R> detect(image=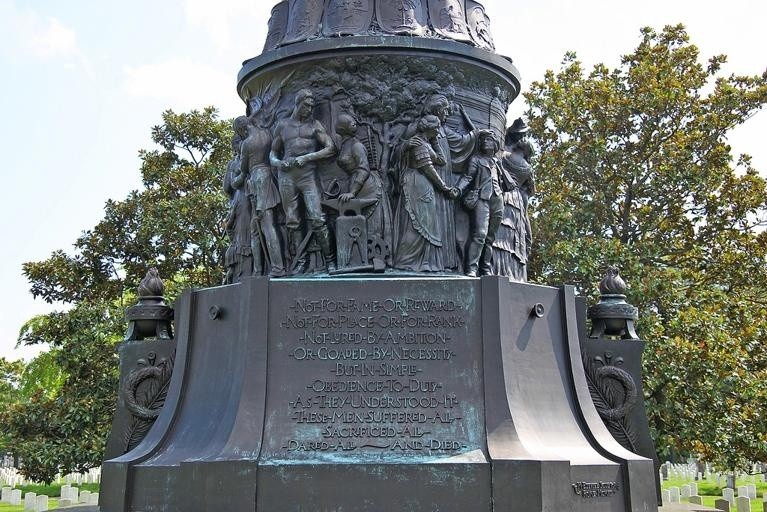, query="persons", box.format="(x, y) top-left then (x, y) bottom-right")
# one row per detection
(221, 89), (536, 283)
